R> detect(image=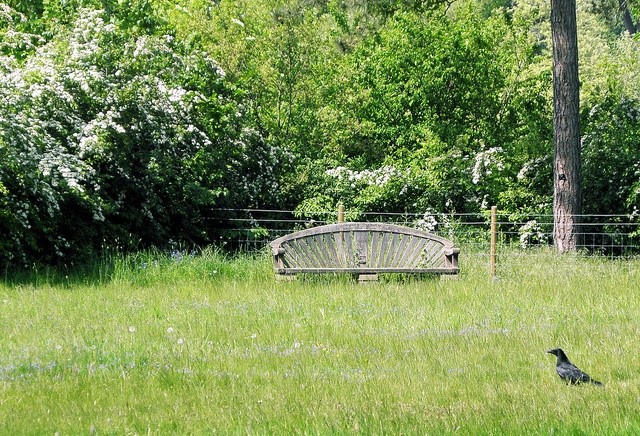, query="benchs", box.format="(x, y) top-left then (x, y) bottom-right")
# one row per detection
(269, 222), (461, 287)
